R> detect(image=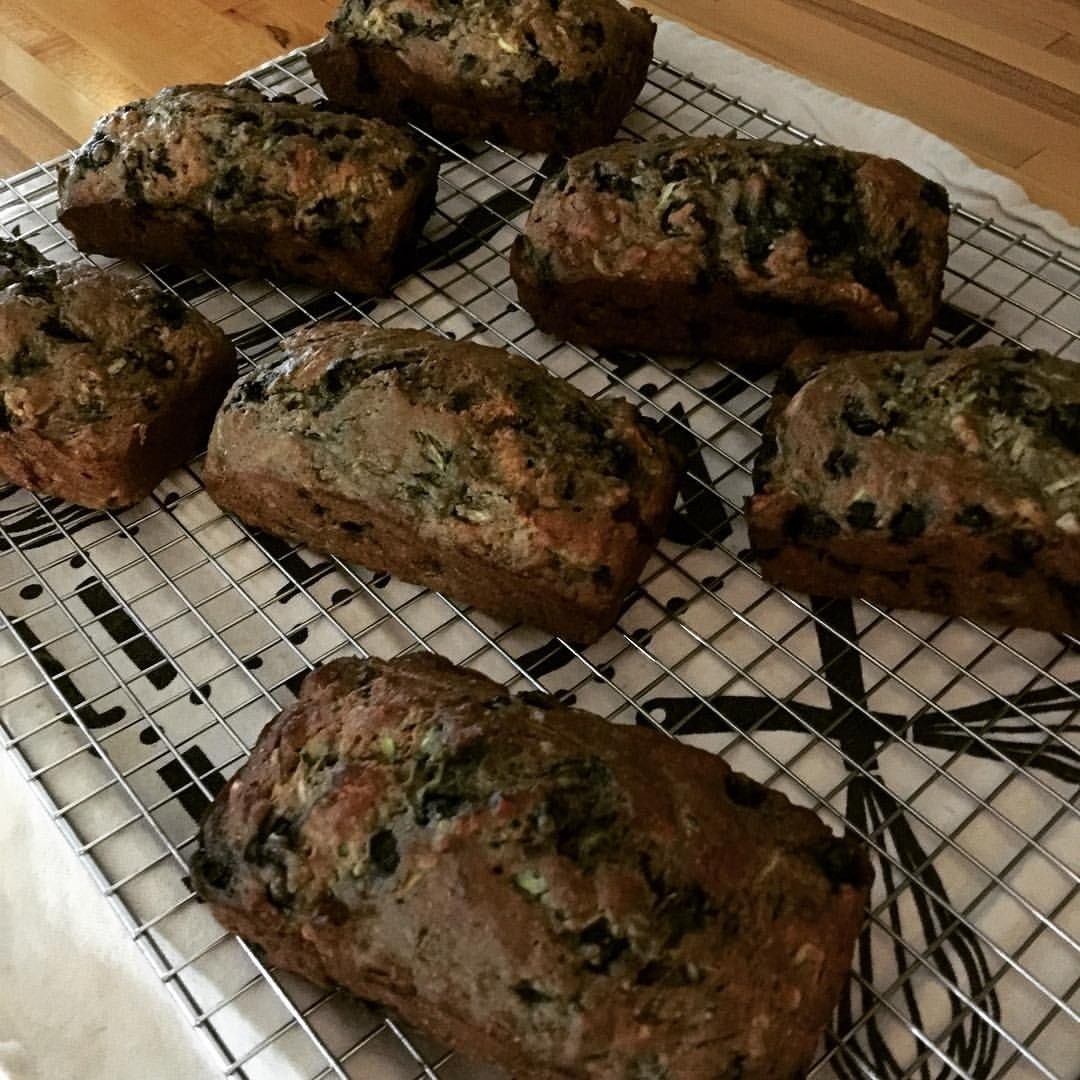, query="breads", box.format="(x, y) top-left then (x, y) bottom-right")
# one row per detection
(0, 0), (1080, 1080)
